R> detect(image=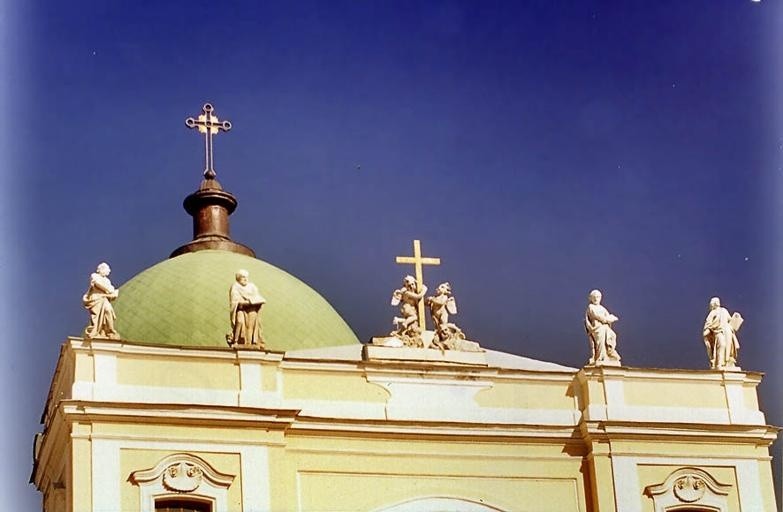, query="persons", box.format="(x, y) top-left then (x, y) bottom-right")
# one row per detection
(80, 257), (121, 341)
(225, 262), (265, 344)
(388, 271), (430, 336)
(700, 295), (745, 371)
(423, 277), (459, 329)
(398, 320), (424, 349)
(429, 323), (481, 352)
(581, 287), (624, 367)
